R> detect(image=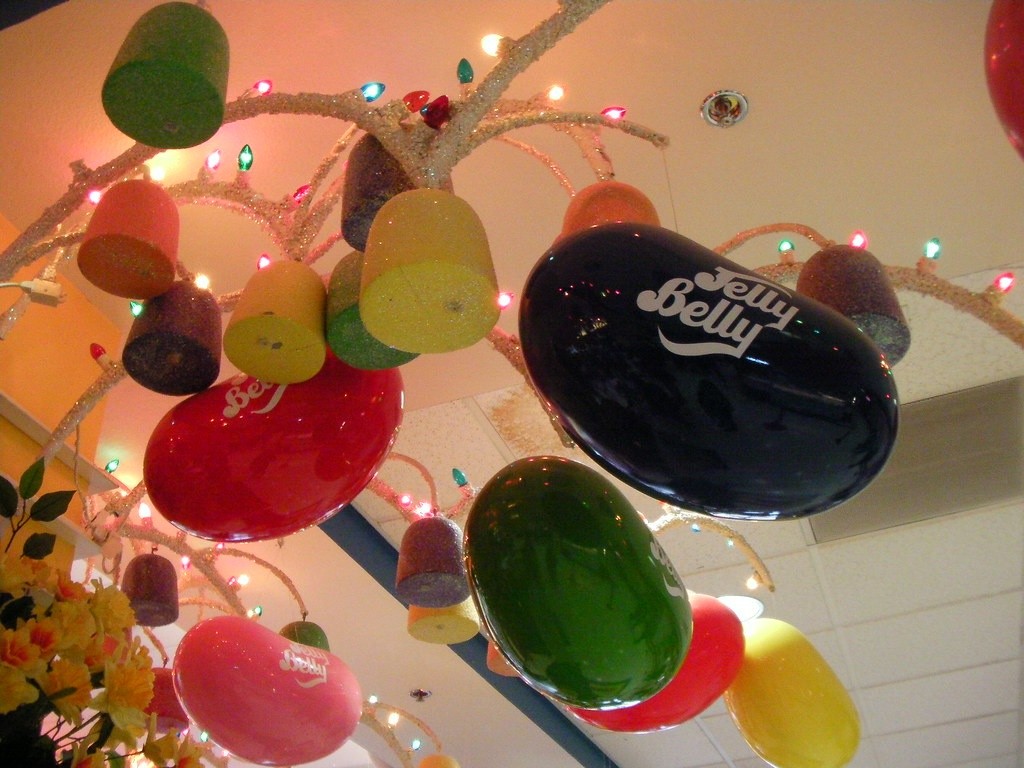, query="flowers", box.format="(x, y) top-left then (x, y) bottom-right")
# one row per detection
(0, 455), (231, 768)
